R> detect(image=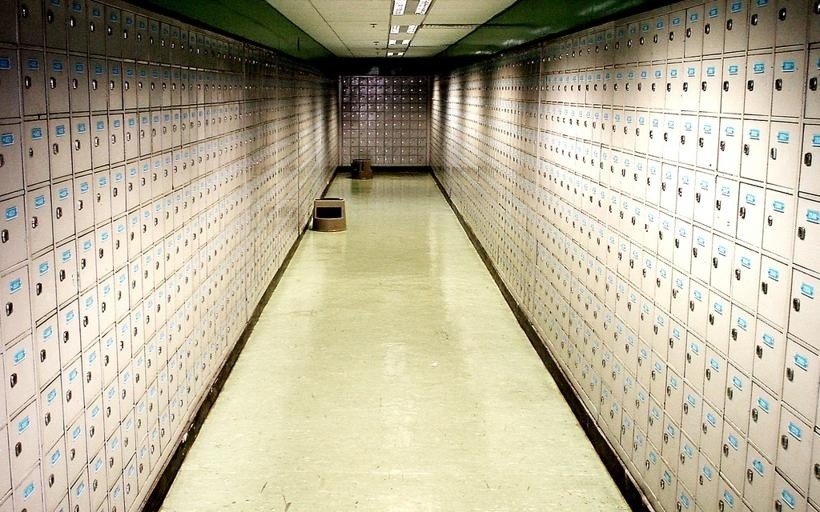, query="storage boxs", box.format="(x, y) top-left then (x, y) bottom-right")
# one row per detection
(340, 75), (429, 167)
(429, 1), (819, 512)
(1, 2), (341, 511)
